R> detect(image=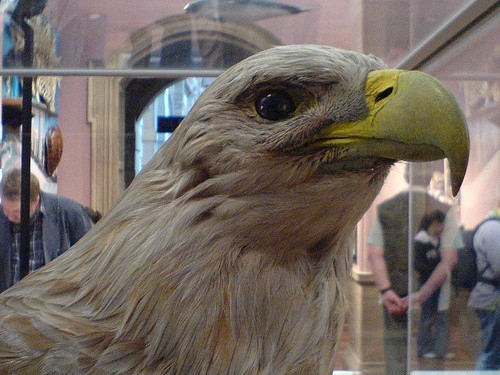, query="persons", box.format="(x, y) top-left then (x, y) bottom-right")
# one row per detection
(0, 168), (93, 293)
(467, 193), (500, 368)
(413, 209), (457, 361)
(367, 162), (464, 375)
(86, 207), (102, 225)
